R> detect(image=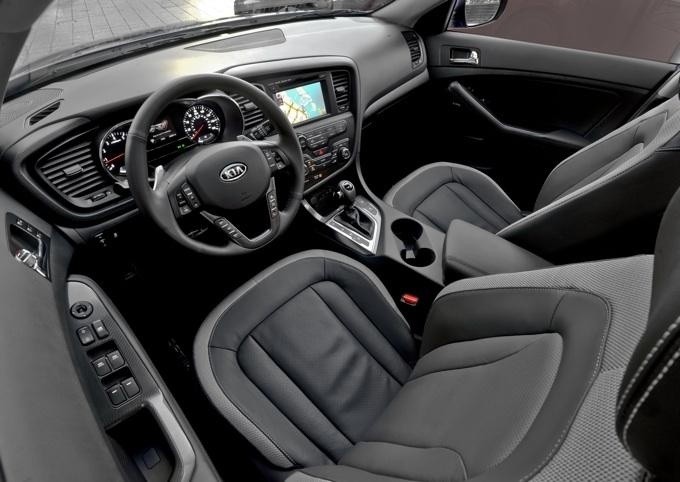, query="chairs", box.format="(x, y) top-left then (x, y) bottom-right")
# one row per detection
(385, 77), (679, 257)
(195, 187), (679, 482)
